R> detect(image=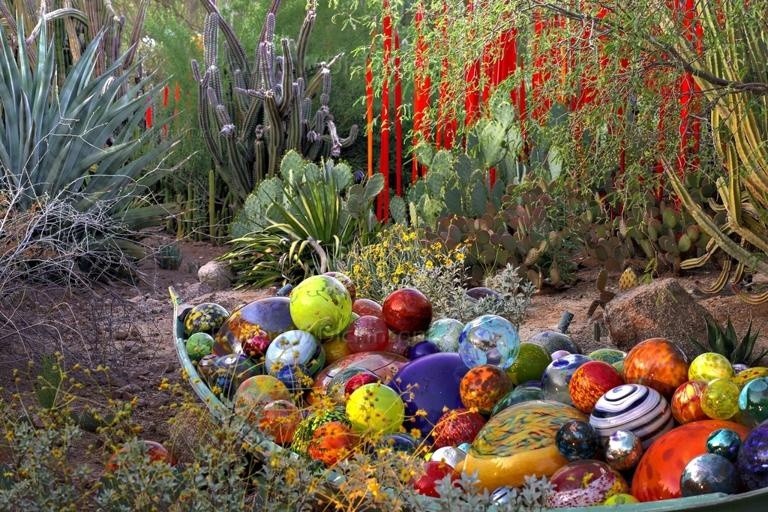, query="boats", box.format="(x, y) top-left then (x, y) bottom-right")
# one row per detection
(165, 285), (766, 510)
